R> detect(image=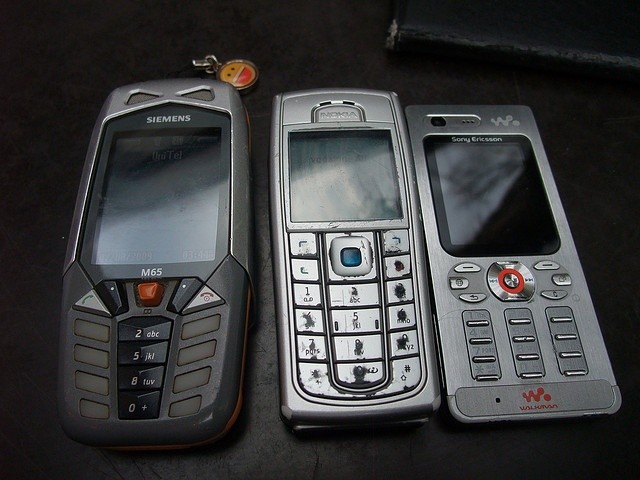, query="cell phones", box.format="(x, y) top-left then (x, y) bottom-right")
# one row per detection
(57, 75), (259, 453)
(269, 88), (442, 442)
(404, 102), (623, 424)
